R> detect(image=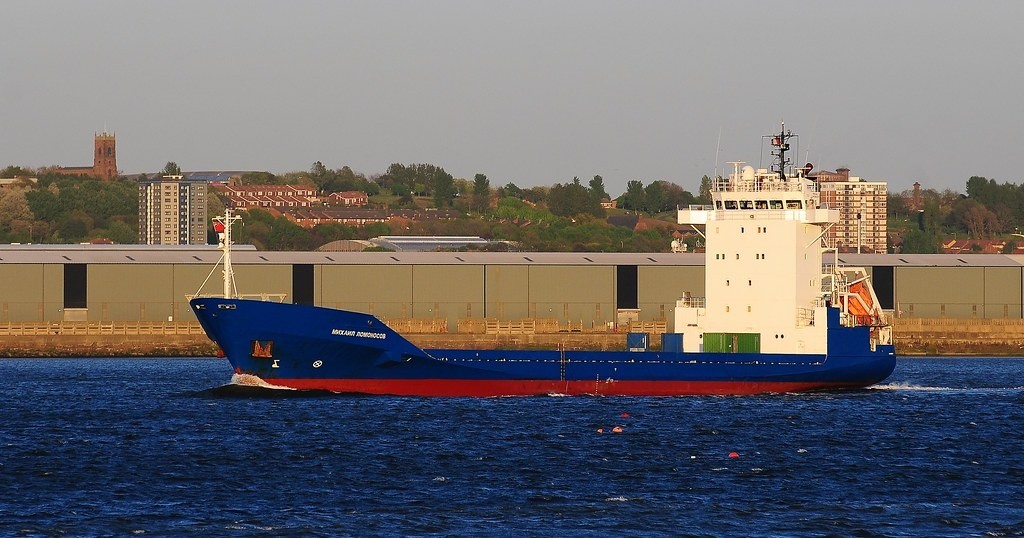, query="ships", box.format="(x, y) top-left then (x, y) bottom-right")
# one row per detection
(187, 119), (899, 396)
